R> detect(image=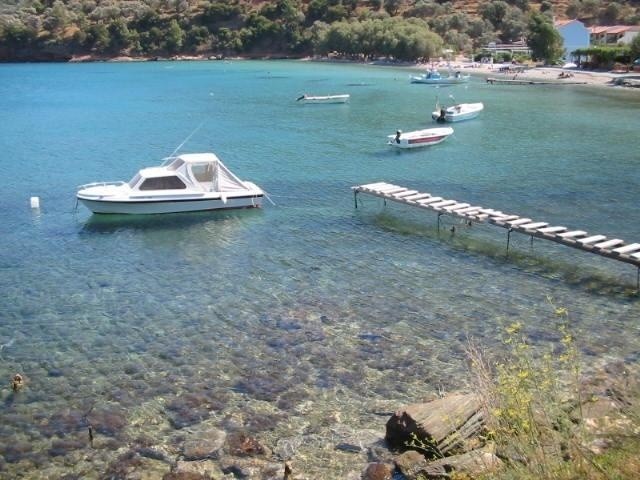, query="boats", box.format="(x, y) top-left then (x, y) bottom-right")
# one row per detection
(431, 102), (485, 123)
(386, 127), (454, 149)
(75, 122), (267, 215)
(410, 62), (473, 85)
(296, 93), (350, 104)
